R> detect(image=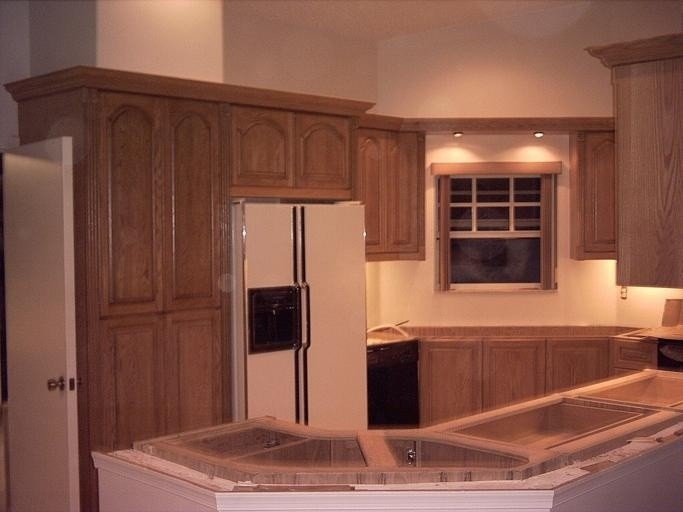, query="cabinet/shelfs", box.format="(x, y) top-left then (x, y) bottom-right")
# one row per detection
(584, 32), (683, 287)
(546, 338), (610, 393)
(610, 339), (657, 377)
(571, 130), (616, 261)
(419, 338), (546, 428)
(357, 126), (425, 261)
